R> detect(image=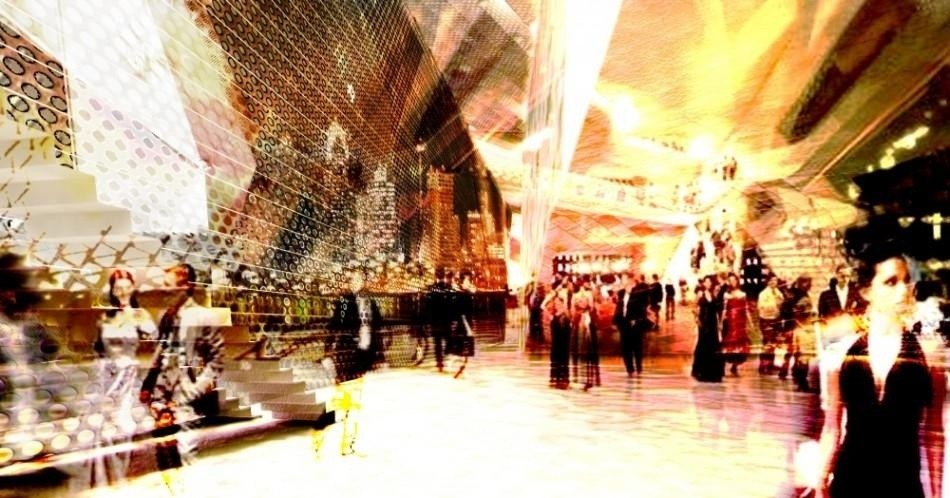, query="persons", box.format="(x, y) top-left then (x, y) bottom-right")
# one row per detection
(76, 267), (159, 493)
(317, 260), (393, 428)
(141, 261), (225, 476)
(801, 245), (944, 497)
(400, 248), (865, 396)
(1, 251), (70, 488)
(906, 258), (949, 342)
